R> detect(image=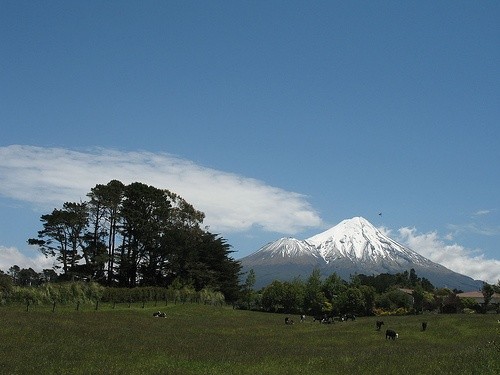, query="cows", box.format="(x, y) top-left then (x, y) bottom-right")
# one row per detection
(377, 320), (384, 330)
(422, 321), (427, 330)
(285, 311), (356, 324)
(386, 329), (399, 340)
(153, 311), (167, 318)
